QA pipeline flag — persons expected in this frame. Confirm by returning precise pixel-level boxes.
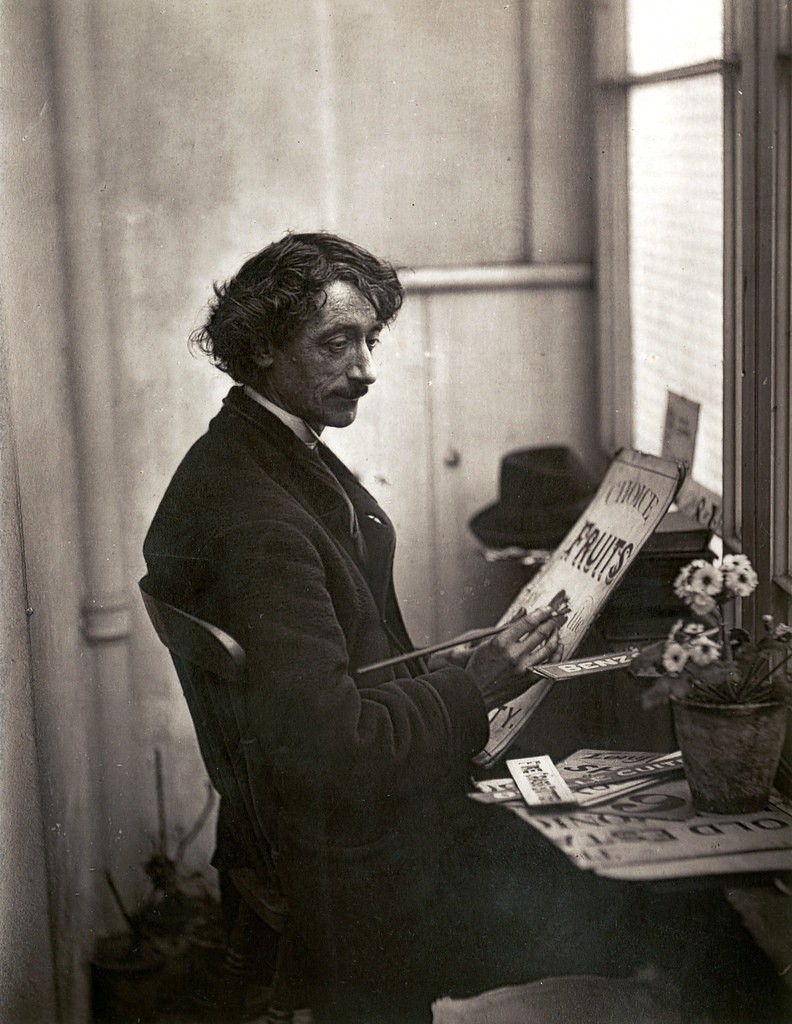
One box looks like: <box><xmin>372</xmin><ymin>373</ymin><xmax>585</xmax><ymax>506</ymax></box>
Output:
<box><xmin>141</xmin><ymin>229</ymin><xmax>792</xmax><ymax>1024</ymax></box>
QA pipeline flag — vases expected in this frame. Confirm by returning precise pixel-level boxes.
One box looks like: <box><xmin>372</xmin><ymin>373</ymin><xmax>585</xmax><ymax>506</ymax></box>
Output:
<box><xmin>668</xmin><ymin>688</ymin><xmax>788</xmax><ymax>815</ymax></box>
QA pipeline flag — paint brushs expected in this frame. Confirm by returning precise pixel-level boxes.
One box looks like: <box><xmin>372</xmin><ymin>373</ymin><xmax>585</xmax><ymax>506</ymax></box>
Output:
<box><xmin>355</xmin><ymin>608</ymin><xmax>573</xmax><ymax>674</ymax></box>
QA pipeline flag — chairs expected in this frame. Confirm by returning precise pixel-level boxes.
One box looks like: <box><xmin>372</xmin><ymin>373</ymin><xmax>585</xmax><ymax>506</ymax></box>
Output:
<box><xmin>138</xmin><ymin>573</ymin><xmax>309</xmax><ymax>1023</ymax></box>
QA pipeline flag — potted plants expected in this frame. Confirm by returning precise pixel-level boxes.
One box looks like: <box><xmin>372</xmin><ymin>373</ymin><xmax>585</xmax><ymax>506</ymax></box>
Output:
<box><xmin>88</xmin><ymin>931</ymin><xmax>168</xmax><ymax>1024</ymax></box>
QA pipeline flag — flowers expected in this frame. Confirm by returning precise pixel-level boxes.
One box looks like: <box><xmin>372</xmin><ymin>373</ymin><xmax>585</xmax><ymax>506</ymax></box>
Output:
<box><xmin>626</xmin><ymin>551</ymin><xmax>792</xmax><ymax>712</ymax></box>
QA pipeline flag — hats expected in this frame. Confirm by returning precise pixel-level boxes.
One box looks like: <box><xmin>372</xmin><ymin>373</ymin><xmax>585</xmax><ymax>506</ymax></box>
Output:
<box><xmin>468</xmin><ymin>445</ymin><xmax>598</xmax><ymax>552</ymax></box>
